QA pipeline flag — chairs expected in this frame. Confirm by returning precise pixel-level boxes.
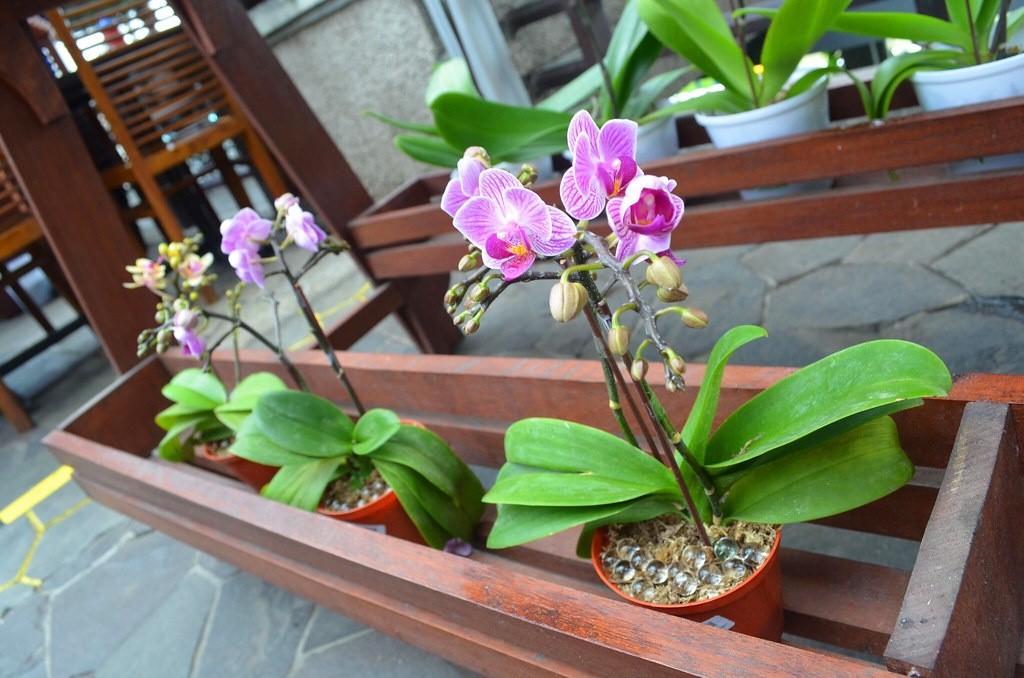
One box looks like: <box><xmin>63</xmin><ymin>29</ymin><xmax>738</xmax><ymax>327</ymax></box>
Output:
<box><xmin>0</xmin><ymin>0</ymin><xmax>289</xmax><ymax>445</ymax></box>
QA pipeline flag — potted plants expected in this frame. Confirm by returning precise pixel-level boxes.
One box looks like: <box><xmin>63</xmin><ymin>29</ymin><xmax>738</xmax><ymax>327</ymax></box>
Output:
<box><xmin>730</xmin><ymin>0</ymin><xmax>1023</xmax><ymax>176</ymax></box>
<box><xmin>636</xmin><ymin>0</ymin><xmax>852</xmax><ymax>198</ymax></box>
<box><xmin>434</xmin><ymin>0</ymin><xmax>703</xmax><ymax>217</ymax></box>
<box><xmin>363</xmin><ymin>52</ymin><xmax>611</xmax><ymax>193</ymax></box>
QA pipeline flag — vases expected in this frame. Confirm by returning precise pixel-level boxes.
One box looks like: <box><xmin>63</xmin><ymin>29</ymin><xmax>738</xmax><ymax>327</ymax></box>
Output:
<box><xmin>584</xmin><ymin>499</ymin><xmax>790</xmax><ymax>634</ymax></box>
<box><xmin>193</xmin><ymin>395</ymin><xmax>278</xmax><ymax>488</ymax></box>
<box><xmin>312</xmin><ymin>410</ymin><xmax>428</xmax><ymax>543</ymax></box>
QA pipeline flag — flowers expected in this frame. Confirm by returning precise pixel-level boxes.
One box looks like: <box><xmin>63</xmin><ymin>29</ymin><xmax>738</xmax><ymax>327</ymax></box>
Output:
<box><xmin>219</xmin><ymin>190</ymin><xmax>491</xmax><ymax>550</ymax></box>
<box><xmin>434</xmin><ymin>116</ymin><xmax>953</xmax><ymax>563</ymax></box>
<box><xmin>121</xmin><ymin>231</ymin><xmax>286</xmax><ymax>457</ymax></box>
